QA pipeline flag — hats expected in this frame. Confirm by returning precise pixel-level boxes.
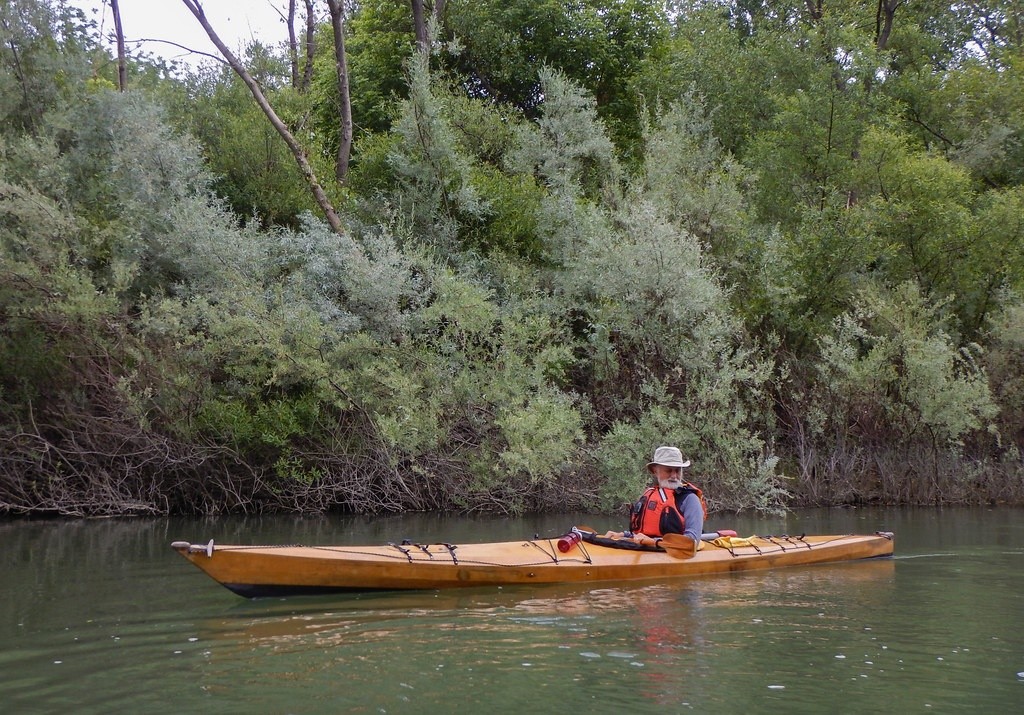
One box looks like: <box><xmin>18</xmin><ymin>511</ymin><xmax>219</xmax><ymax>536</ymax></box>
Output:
<box><xmin>646</xmin><ymin>446</ymin><xmax>691</xmax><ymax>474</ymax></box>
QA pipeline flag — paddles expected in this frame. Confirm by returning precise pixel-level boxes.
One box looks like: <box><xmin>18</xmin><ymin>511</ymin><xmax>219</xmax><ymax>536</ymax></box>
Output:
<box><xmin>573</xmin><ymin>524</ymin><xmax>697</xmax><ymax>562</ymax></box>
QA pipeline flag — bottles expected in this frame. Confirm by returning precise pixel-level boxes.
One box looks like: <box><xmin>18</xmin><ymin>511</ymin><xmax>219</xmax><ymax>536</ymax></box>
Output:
<box><xmin>557</xmin><ymin>526</ymin><xmax>583</xmax><ymax>553</ymax></box>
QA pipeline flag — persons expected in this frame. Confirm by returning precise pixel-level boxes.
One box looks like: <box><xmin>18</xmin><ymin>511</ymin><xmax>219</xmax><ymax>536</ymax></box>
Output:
<box><xmin>605</xmin><ymin>446</ymin><xmax>704</xmax><ymax>549</ymax></box>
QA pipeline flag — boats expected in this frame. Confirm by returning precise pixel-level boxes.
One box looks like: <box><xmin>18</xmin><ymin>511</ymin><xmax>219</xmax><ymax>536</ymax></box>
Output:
<box><xmin>171</xmin><ymin>532</ymin><xmax>896</xmax><ymax>599</ymax></box>
